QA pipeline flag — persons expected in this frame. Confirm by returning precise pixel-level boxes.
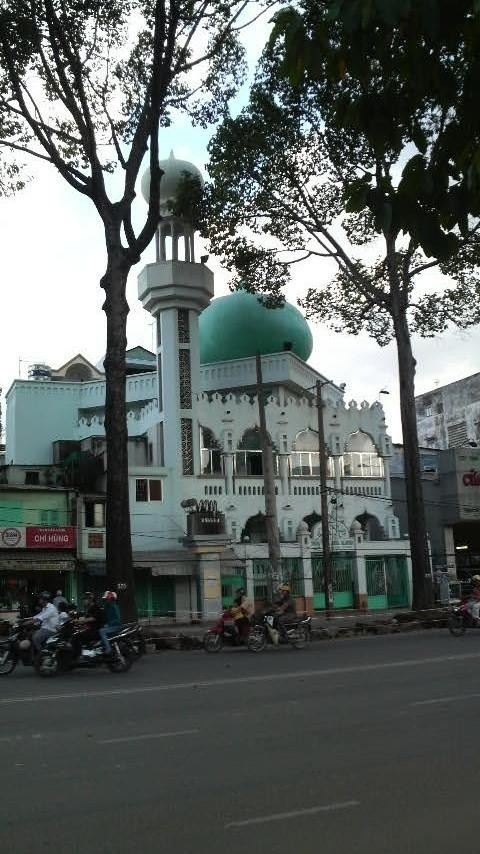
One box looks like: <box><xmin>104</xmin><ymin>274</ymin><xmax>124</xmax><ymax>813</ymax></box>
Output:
<box><xmin>230</xmin><ymin>589</ymin><xmax>254</xmax><ymax>638</ymax></box>
<box><xmin>272</xmin><ymin>582</ymin><xmax>297</xmax><ymax>642</ymax></box>
<box><xmin>468</xmin><ymin>575</ymin><xmax>480</xmax><ymax>622</ymax></box>
<box><xmin>24</xmin><ymin>590</ymin><xmax>122</xmax><ymax>655</ymax></box>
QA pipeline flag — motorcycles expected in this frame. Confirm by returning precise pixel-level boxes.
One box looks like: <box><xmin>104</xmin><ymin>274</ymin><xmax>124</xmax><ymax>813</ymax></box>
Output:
<box><xmin>202</xmin><ymin>605</ymin><xmax>311</xmax><ymax>653</ymax></box>
<box><xmin>0</xmin><ymin>612</ymin><xmax>144</xmax><ymax>676</ymax></box>
<box><xmin>448</xmin><ymin>597</ymin><xmax>480</xmax><ymax>635</ymax></box>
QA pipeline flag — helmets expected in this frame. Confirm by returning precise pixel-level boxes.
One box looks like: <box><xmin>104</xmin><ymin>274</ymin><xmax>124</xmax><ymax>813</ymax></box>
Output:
<box><xmin>37</xmin><ymin>592</ymin><xmax>51</xmax><ymax>601</ymax></box>
<box><xmin>102</xmin><ymin>591</ymin><xmax>117</xmax><ymax>600</ymax></box>
<box><xmin>472</xmin><ymin>575</ymin><xmax>479</xmax><ymax>585</ymax></box>
<box><xmin>236</xmin><ymin>588</ymin><xmax>245</xmax><ymax>595</ymax></box>
<box><xmin>279</xmin><ymin>585</ymin><xmax>289</xmax><ymax>592</ymax></box>
